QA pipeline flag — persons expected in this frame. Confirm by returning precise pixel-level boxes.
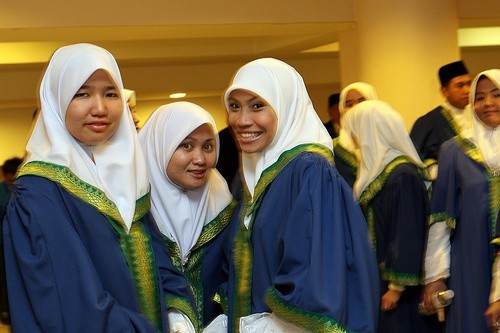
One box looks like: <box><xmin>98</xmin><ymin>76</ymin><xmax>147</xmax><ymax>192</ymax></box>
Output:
<box><xmin>202</xmin><ymin>58</ymin><xmax>381</xmax><ymax>333</ymax></box>
<box><xmin>137</xmin><ymin>101</ymin><xmax>237</xmax><ymax>333</ymax></box>
<box><xmin>123</xmin><ymin>88</ymin><xmax>140</xmax><ymax>130</ymax></box>
<box><xmin>216</xmin><ymin>127</ymin><xmax>239</xmax><ymax>192</ymax></box>
<box><xmin>324</xmin><ymin>93</ymin><xmax>340</xmax><ymax>139</ymax></box>
<box><xmin>332</xmin><ymin>82</ymin><xmax>437</xmax><ymax>333</ymax></box>
<box><xmin>2</xmin><ymin>43</ymin><xmax>169</xmax><ymax>333</ymax></box>
<box><xmin>409</xmin><ymin>61</ymin><xmax>472</xmax><ymax>162</ymax></box>
<box><xmin>0</xmin><ymin>157</ymin><xmax>24</xmax><ymax>322</ymax></box>
<box><xmin>422</xmin><ymin>69</ymin><xmax>500</xmax><ymax>333</ymax></box>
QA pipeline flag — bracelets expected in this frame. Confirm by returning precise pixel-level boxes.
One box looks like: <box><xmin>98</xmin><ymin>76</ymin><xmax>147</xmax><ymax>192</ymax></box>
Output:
<box><xmin>388</xmin><ymin>284</ymin><xmax>404</xmax><ymax>291</ymax></box>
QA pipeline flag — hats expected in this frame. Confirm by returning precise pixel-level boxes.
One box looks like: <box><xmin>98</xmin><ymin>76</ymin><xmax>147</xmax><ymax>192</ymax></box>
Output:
<box><xmin>328</xmin><ymin>94</ymin><xmax>339</xmax><ymax>106</ymax></box>
<box><xmin>439</xmin><ymin>60</ymin><xmax>467</xmax><ymax>84</ymax></box>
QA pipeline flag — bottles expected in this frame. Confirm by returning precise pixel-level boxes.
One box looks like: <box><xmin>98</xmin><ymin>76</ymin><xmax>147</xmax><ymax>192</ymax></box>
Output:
<box><xmin>416</xmin><ymin>289</ymin><xmax>455</xmax><ymax>314</ymax></box>
